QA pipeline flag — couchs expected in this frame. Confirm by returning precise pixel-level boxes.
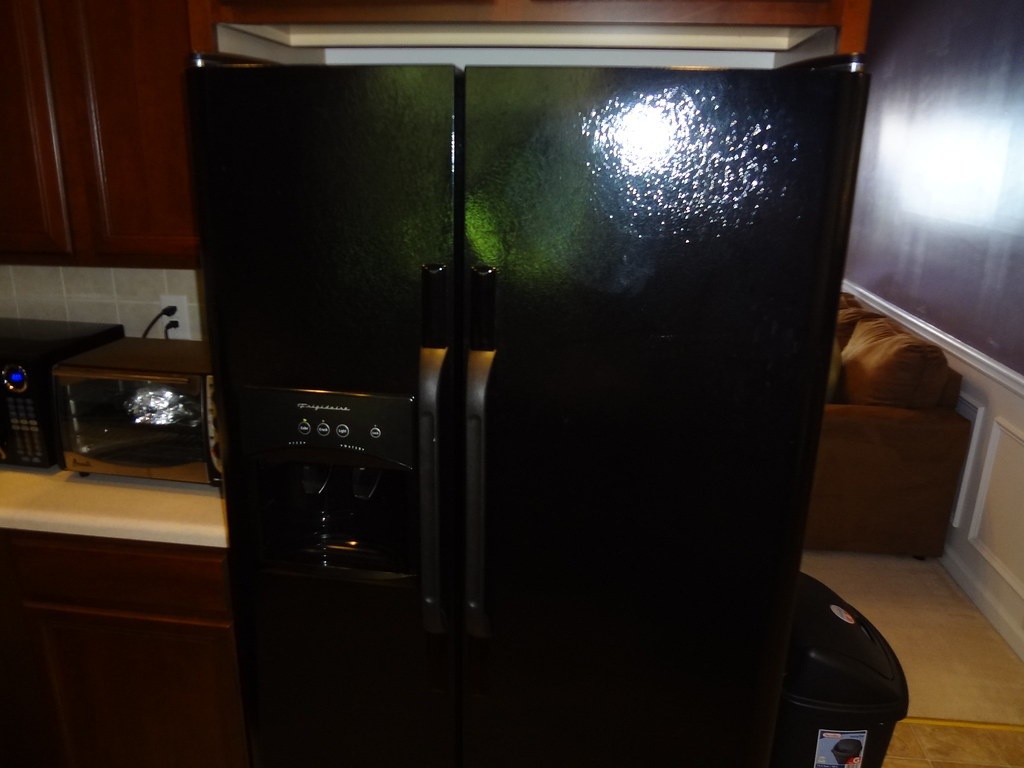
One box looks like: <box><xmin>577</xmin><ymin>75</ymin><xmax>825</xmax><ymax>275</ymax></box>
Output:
<box><xmin>805</xmin><ymin>293</ymin><xmax>977</xmax><ymax>557</ymax></box>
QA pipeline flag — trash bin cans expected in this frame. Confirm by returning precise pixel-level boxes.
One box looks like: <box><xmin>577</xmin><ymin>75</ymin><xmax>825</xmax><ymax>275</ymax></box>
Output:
<box><xmin>767</xmin><ymin>572</ymin><xmax>909</xmax><ymax>768</ymax></box>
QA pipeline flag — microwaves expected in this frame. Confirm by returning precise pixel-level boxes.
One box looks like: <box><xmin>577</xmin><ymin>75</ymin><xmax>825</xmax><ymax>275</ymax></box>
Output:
<box><xmin>0</xmin><ymin>317</ymin><xmax>125</xmax><ymax>471</ymax></box>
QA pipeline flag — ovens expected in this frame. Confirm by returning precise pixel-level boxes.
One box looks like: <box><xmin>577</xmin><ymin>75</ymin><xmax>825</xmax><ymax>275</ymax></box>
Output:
<box><xmin>51</xmin><ymin>336</ymin><xmax>223</xmax><ymax>486</ymax></box>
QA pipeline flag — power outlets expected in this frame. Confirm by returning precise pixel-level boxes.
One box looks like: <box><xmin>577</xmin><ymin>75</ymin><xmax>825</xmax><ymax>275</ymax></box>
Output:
<box><xmin>160</xmin><ymin>295</ymin><xmax>191</xmax><ymax>339</ymax></box>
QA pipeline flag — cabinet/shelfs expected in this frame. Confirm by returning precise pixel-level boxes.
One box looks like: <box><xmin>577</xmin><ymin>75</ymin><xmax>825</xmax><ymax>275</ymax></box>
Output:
<box><xmin>0</xmin><ymin>529</ymin><xmax>249</xmax><ymax>768</ymax></box>
<box><xmin>0</xmin><ymin>0</ymin><xmax>213</xmax><ymax>270</ymax></box>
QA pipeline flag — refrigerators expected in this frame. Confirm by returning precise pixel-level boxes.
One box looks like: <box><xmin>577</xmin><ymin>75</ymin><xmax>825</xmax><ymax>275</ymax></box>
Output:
<box><xmin>182</xmin><ymin>50</ymin><xmax>873</xmax><ymax>768</ymax></box>
